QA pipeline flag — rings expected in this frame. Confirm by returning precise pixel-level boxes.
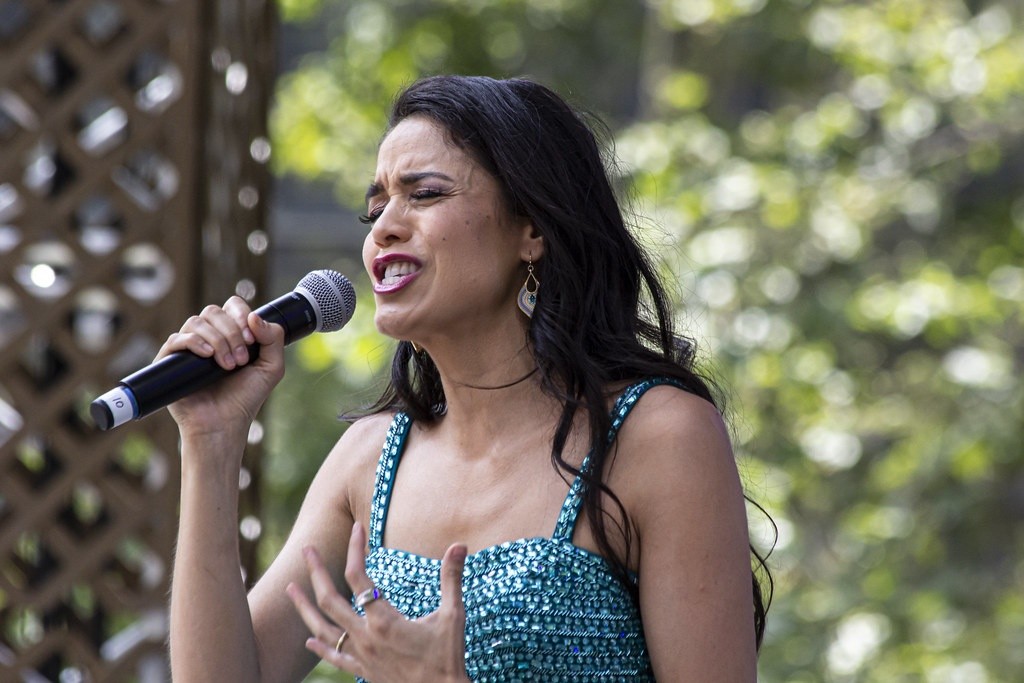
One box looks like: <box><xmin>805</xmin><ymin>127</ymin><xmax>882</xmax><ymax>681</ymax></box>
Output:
<box><xmin>356</xmin><ymin>587</ymin><xmax>382</xmax><ymax>607</ymax></box>
<box><xmin>335</xmin><ymin>631</ymin><xmax>347</xmax><ymax>653</ymax></box>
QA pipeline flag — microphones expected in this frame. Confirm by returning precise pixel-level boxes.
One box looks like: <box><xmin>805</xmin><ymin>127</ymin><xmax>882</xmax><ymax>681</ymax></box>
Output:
<box><xmin>91</xmin><ymin>268</ymin><xmax>357</xmax><ymax>431</ymax></box>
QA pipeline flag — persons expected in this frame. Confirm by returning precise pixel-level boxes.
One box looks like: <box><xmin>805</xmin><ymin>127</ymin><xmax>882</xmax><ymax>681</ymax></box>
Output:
<box><xmin>154</xmin><ymin>73</ymin><xmax>778</xmax><ymax>683</ymax></box>
